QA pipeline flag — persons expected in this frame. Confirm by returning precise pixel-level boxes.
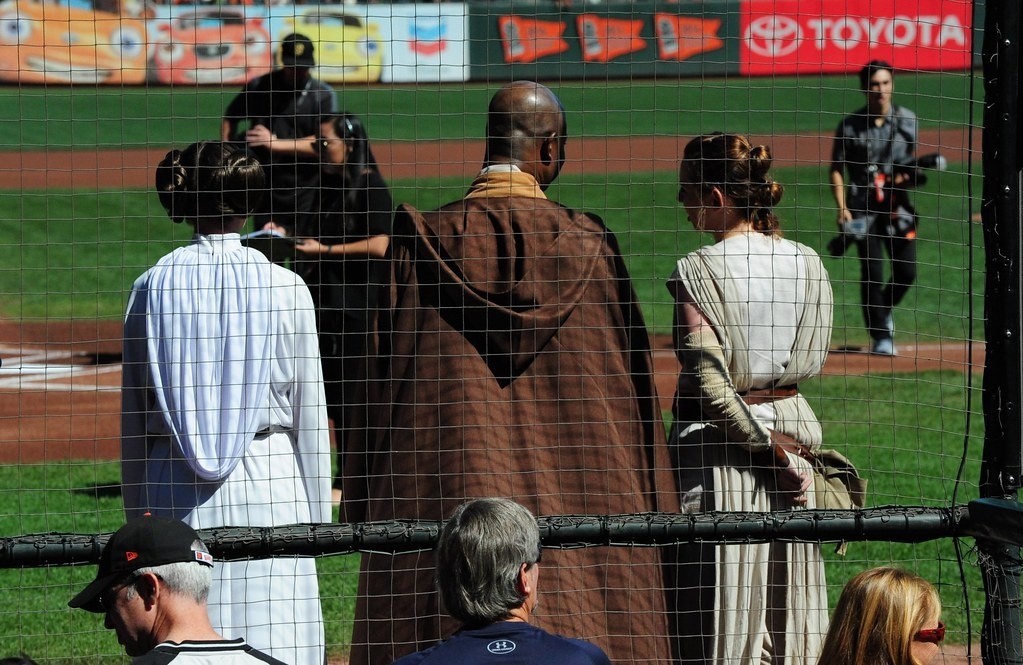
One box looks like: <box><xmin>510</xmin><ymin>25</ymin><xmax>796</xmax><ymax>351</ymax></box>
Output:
<box><xmin>68</xmin><ymin>512</ymin><xmax>285</xmax><ymax>665</ymax></box>
<box><xmin>339</xmin><ymin>81</ymin><xmax>680</xmax><ymax>665</ymax></box>
<box><xmin>819</xmin><ymin>567</ymin><xmax>942</xmax><ymax>665</ymax></box>
<box><xmin>220</xmin><ymin>33</ymin><xmax>335</xmax><ymax>238</ymax></box>
<box><xmin>666</xmin><ymin>130</ymin><xmax>834</xmax><ymax>665</ymax></box>
<box><xmin>265</xmin><ymin>113</ymin><xmax>393</xmax><ymax>524</ymax></box>
<box><xmin>120</xmin><ymin>141</ymin><xmax>331</xmax><ymax>665</ymax></box>
<box><xmin>830</xmin><ymin>59</ymin><xmax>920</xmax><ymax>357</ymax></box>
<box><xmin>393</xmin><ymin>496</ymin><xmax>613</xmax><ymax>664</ymax></box>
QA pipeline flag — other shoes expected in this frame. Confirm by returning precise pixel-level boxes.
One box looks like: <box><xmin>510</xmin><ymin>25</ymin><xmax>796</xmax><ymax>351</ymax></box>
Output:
<box><xmin>886</xmin><ymin>312</ymin><xmax>897</xmax><ymax>334</ymax></box>
<box><xmin>875</xmin><ymin>341</ymin><xmax>897</xmax><ymax>358</ymax></box>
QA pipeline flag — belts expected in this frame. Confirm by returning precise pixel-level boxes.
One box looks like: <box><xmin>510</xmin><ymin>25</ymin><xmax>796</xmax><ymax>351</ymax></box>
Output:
<box><xmin>741</xmin><ymin>383</ymin><xmax>798</xmax><ymax>404</ymax></box>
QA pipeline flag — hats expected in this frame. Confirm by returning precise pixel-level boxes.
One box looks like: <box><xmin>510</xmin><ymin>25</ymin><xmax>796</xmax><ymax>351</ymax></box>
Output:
<box><xmin>67</xmin><ymin>512</ymin><xmax>215</xmax><ymax>613</ymax></box>
<box><xmin>281</xmin><ymin>34</ymin><xmax>317</xmax><ymax>67</ymax></box>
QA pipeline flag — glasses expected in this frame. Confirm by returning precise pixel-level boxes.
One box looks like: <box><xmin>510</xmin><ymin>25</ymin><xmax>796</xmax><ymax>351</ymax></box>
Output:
<box><xmin>914</xmin><ymin>620</ymin><xmax>945</xmax><ymax>643</ymax></box>
<box><xmin>526</xmin><ymin>543</ymin><xmax>544</xmax><ymax>573</ymax></box>
<box><xmin>309</xmin><ymin>140</ymin><xmax>332</xmax><ymax>156</ymax></box>
<box><xmin>96</xmin><ymin>573</ymin><xmax>163</xmax><ymax>612</ymax></box>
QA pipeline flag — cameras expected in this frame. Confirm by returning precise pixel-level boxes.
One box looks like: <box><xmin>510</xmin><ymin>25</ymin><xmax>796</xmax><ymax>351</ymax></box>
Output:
<box><xmin>828</xmin><ymin>218</ymin><xmax>868</xmax><ymax>259</ymax></box>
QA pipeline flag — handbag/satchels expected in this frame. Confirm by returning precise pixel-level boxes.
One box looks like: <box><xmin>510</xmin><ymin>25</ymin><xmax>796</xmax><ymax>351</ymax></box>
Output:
<box><xmin>814</xmin><ymin>449</ymin><xmax>868</xmax><ymax>556</ymax></box>
